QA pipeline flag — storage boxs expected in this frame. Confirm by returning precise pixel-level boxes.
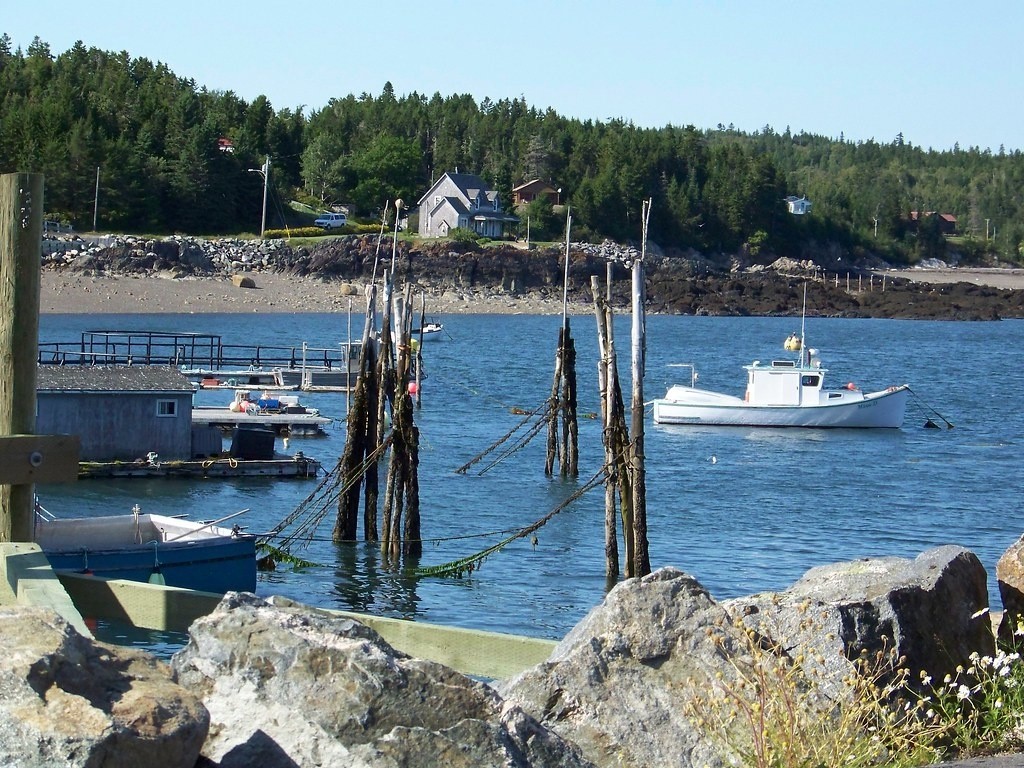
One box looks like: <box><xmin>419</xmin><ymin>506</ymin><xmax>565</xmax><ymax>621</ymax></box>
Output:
<box><xmin>258</xmin><ymin>396</ymin><xmax>307</xmax><ymax>415</ymax></box>
<box><xmin>202</xmin><ymin>379</ymin><xmax>221</xmax><ymax>385</ymax></box>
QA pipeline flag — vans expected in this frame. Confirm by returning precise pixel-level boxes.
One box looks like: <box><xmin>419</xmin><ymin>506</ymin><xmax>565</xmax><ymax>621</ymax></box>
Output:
<box><xmin>314</xmin><ymin>213</ymin><xmax>347</xmax><ymax>230</ymax></box>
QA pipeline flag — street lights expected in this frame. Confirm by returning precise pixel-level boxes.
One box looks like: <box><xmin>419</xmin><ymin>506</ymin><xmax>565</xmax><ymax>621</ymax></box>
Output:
<box><xmin>247</xmin><ymin>168</ymin><xmax>269</xmax><ymax>237</ymax></box>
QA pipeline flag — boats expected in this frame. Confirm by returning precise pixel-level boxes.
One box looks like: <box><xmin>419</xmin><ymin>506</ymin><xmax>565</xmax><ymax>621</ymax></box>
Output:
<box><xmin>36</xmin><ymin>503</ymin><xmax>258</xmax><ymax>595</ymax></box>
<box><xmin>652</xmin><ymin>281</ymin><xmax>911</xmax><ymax>430</ymax></box>
<box><xmin>376</xmin><ymin>316</ymin><xmax>445</xmax><ymax>343</ymax></box>
<box><xmin>182</xmin><ymin>335</ymin><xmax>430</xmax><ymax>436</ymax></box>
